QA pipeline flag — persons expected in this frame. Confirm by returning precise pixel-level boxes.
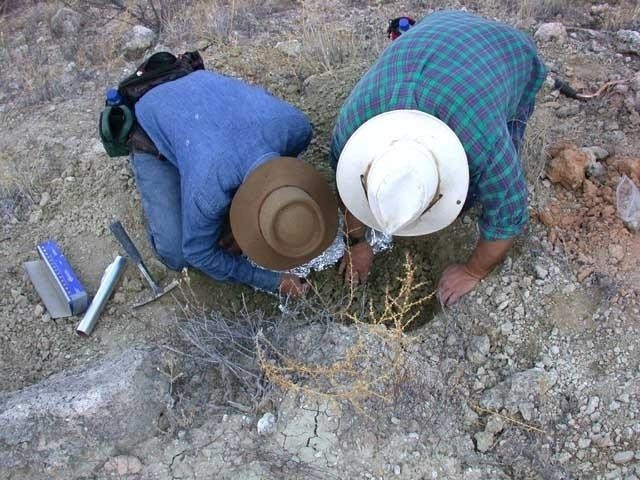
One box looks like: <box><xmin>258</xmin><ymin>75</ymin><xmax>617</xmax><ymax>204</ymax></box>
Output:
<box><xmin>329</xmin><ymin>9</ymin><xmax>545</xmax><ymax>307</ymax></box>
<box><xmin>126</xmin><ymin>53</ymin><xmax>315</xmax><ymax>301</ymax></box>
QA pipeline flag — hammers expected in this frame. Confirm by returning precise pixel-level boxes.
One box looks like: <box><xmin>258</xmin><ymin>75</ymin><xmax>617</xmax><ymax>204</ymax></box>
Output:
<box><xmin>109</xmin><ymin>221</ymin><xmax>179</xmax><ymax>309</ymax></box>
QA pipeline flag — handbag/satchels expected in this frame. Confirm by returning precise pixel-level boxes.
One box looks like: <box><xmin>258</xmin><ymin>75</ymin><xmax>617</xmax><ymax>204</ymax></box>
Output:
<box><xmin>98</xmin><ymin>49</ymin><xmax>205</xmax><ymax>162</ymax></box>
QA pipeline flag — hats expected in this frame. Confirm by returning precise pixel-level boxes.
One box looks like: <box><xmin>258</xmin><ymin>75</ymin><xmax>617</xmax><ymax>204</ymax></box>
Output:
<box><xmin>229</xmin><ymin>156</ymin><xmax>339</xmax><ymax>271</ymax></box>
<box><xmin>335</xmin><ymin>109</ymin><xmax>470</xmax><ymax>237</ymax></box>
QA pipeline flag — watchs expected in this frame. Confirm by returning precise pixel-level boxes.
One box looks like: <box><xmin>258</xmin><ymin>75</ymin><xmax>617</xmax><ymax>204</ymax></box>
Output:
<box><xmin>343</xmin><ymin>232</ymin><xmax>366</xmax><ymax>247</ymax></box>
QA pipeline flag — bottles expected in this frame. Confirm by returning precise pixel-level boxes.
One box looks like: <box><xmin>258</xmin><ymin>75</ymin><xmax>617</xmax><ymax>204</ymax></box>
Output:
<box><xmin>398</xmin><ymin>18</ymin><xmax>413</xmax><ymax>35</ymax></box>
<box><xmin>106</xmin><ymin>90</ymin><xmax>122</xmax><ymax>105</ymax></box>
